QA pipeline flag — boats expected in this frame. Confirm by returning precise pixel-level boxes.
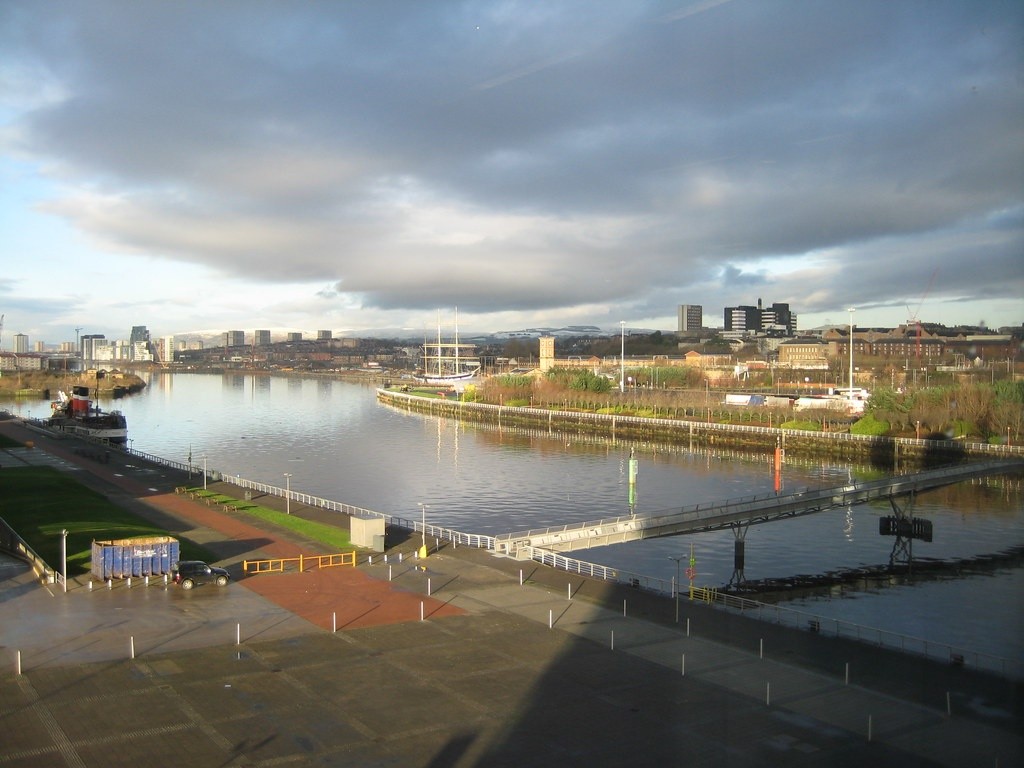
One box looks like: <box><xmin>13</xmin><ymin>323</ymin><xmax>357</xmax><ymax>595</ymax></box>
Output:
<box><xmin>42</xmin><ymin>371</ymin><xmax>128</xmax><ymax>444</ymax></box>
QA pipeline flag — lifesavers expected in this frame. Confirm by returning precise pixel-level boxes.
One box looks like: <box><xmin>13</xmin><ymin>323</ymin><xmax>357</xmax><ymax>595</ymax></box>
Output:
<box><xmin>685</xmin><ymin>568</ymin><xmax>695</xmax><ymax>579</ymax></box>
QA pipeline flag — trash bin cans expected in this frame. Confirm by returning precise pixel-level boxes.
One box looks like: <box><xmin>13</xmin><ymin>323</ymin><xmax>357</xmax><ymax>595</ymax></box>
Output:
<box><xmin>245</xmin><ymin>491</ymin><xmax>252</xmax><ymax>502</ymax></box>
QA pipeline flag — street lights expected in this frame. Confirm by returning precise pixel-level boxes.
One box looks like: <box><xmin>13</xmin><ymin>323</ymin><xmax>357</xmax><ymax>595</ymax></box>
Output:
<box><xmin>666</xmin><ymin>554</ymin><xmax>687</xmax><ymax>623</ymax></box>
<box><xmin>847</xmin><ymin>307</ymin><xmax>856</xmax><ymax>403</ymax></box>
<box><xmin>283</xmin><ymin>472</ymin><xmax>292</xmax><ymax>516</ymax></box>
<box><xmin>417</xmin><ymin>503</ymin><xmax>430</xmax><ymax>546</ymax></box>
<box><xmin>619</xmin><ymin>320</ymin><xmax>627</xmax><ymax>393</ymax></box>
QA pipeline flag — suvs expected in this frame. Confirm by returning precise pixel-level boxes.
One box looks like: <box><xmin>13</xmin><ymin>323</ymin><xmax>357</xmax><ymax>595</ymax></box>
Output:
<box><xmin>170</xmin><ymin>560</ymin><xmax>231</xmax><ymax>591</ymax></box>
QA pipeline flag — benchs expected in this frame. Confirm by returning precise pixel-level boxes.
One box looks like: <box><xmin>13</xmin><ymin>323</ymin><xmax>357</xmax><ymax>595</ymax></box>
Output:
<box><xmin>223</xmin><ymin>505</ymin><xmax>238</xmax><ymax>513</ymax></box>
<box><xmin>174</xmin><ymin>486</ymin><xmax>187</xmax><ymax>494</ymax></box>
<box><xmin>206</xmin><ymin>498</ymin><xmax>219</xmax><ymax>506</ymax></box>
<box><xmin>189</xmin><ymin>492</ymin><xmax>203</xmax><ymax>500</ymax></box>
